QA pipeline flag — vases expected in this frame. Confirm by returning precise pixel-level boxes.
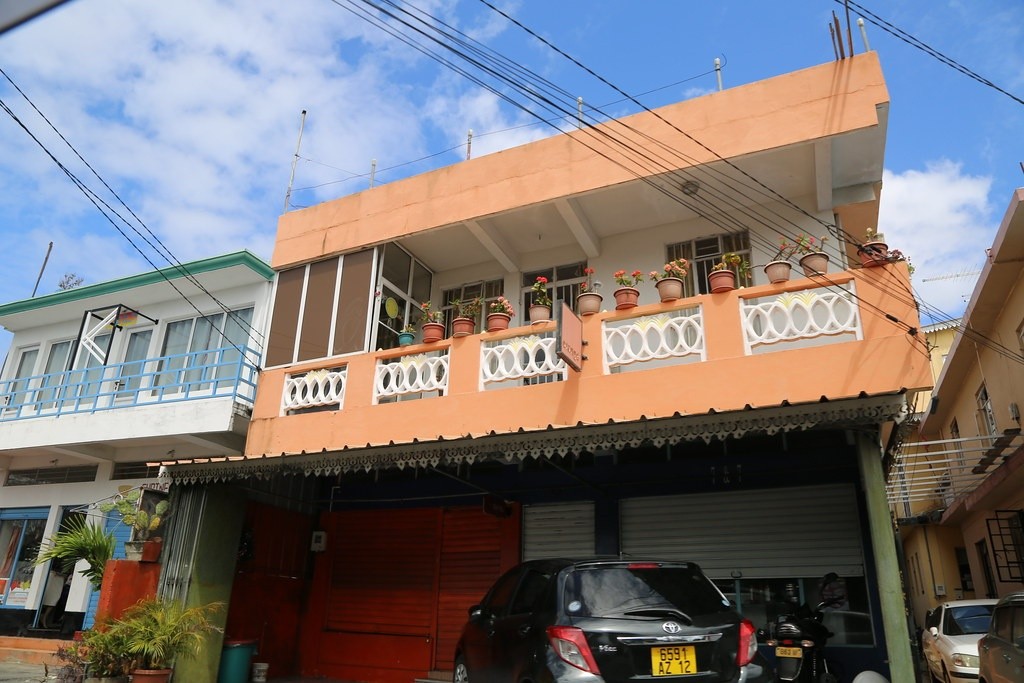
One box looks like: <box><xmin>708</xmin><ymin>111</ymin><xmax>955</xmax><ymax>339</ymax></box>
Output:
<box><xmin>613</xmin><ymin>287</ymin><xmax>640</xmax><ymax>310</ymax></box>
<box><xmin>487</xmin><ymin>313</ymin><xmax>511</xmax><ymax>332</ymax></box>
<box><xmin>764</xmin><ymin>261</ymin><xmax>792</xmax><ymax>284</ymax></box>
<box><xmin>577</xmin><ymin>293</ymin><xmax>604</xmax><ymax>316</ymax></box>
<box><xmin>857</xmin><ymin>242</ymin><xmax>888</xmax><ymax>269</ymax></box>
<box><xmin>799</xmin><ymin>252</ymin><xmax>830</xmax><ymax>278</ymax></box>
<box><xmin>708</xmin><ymin>270</ymin><xmax>735</xmax><ymax>294</ymax></box>
<box><xmin>529</xmin><ymin>305</ymin><xmax>551</xmax><ymax>326</ymax></box>
<box><xmin>452</xmin><ymin>316</ymin><xmax>476</xmax><ymax>339</ymax></box>
<box><xmin>422</xmin><ymin>324</ymin><xmax>445</xmax><ymax>344</ymax></box>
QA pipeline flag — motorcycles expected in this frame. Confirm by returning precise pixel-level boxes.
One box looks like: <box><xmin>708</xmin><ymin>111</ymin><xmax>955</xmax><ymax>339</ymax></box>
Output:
<box><xmin>766</xmin><ymin>606</ymin><xmax>832</xmax><ymax>681</ymax></box>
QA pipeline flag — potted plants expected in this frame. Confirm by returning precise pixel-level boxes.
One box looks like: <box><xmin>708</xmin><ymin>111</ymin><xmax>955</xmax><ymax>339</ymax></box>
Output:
<box><xmin>398</xmin><ymin>324</ymin><xmax>417</xmax><ymax>348</ymax></box>
<box><xmin>100</xmin><ymin>491</ymin><xmax>172</xmax><ymax>562</ymax></box>
<box><xmin>50</xmin><ymin>593</ymin><xmax>228</xmax><ymax>683</ymax></box>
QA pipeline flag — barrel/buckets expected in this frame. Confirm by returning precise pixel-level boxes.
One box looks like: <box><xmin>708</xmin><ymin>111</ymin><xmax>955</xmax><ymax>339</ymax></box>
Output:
<box><xmin>252</xmin><ymin>662</ymin><xmax>269</xmax><ymax>682</ymax></box>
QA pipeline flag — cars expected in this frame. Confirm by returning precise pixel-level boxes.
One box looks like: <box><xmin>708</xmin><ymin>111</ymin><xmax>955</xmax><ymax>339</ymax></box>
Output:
<box><xmin>921</xmin><ymin>598</ymin><xmax>1001</xmax><ymax>683</ymax></box>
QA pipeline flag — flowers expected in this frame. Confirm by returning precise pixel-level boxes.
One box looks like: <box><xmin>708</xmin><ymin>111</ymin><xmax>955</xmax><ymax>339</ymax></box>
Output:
<box><xmin>711</xmin><ymin>253</ymin><xmax>741</xmax><ymax>271</ymax></box>
<box><xmin>581</xmin><ymin>267</ymin><xmax>602</xmax><ymax>294</ymax></box>
<box><xmin>421</xmin><ymin>301</ymin><xmax>445</xmax><ymax>323</ymax></box>
<box><xmin>800</xmin><ymin>235</ymin><xmax>829</xmax><ymax>255</ymax></box>
<box><xmin>655</xmin><ymin>277</ymin><xmax>683</xmax><ymax>303</ymax></box>
<box><xmin>488</xmin><ymin>296</ymin><xmax>516</xmax><ymax>317</ymax></box>
<box><xmin>448</xmin><ymin>297</ymin><xmax>484</xmax><ymax>321</ymax></box>
<box><xmin>530</xmin><ymin>277</ymin><xmax>553</xmax><ymax>309</ymax></box>
<box><xmin>613</xmin><ymin>270</ymin><xmax>644</xmax><ymax>288</ymax></box>
<box><xmin>865</xmin><ymin>228</ymin><xmax>885</xmax><ymax>243</ymax></box>
<box><xmin>769</xmin><ymin>234</ymin><xmax>805</xmax><ymax>262</ymax></box>
<box><xmin>649</xmin><ymin>258</ymin><xmax>690</xmax><ymax>281</ymax></box>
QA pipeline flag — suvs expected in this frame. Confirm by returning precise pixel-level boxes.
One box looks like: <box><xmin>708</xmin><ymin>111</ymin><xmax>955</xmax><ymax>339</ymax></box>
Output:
<box><xmin>450</xmin><ymin>551</ymin><xmax>758</xmax><ymax>683</ymax></box>
<box><xmin>977</xmin><ymin>591</ymin><xmax>1024</xmax><ymax>683</ymax></box>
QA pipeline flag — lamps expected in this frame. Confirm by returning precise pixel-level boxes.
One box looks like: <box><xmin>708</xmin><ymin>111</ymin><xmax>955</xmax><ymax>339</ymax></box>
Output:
<box><xmin>682</xmin><ymin>181</ymin><xmax>700</xmax><ymax>196</ymax></box>
